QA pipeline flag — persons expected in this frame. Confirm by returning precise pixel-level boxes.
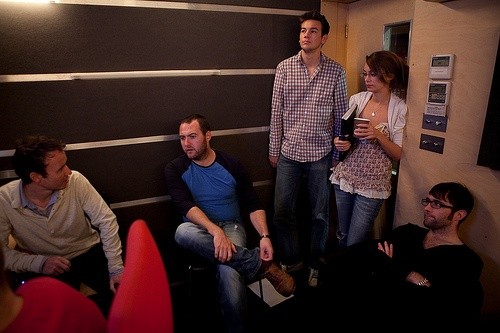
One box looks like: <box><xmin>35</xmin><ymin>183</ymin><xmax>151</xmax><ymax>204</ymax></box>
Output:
<box><xmin>334</xmin><ymin>182</ymin><xmax>482</xmax><ymax>333</ymax></box>
<box><xmin>162</xmin><ymin>108</ymin><xmax>294</xmax><ymax>332</ymax></box>
<box><xmin>0</xmin><ymin>128</ymin><xmax>126</xmax><ymax>311</ymax></box>
<box><xmin>326</xmin><ymin>50</ymin><xmax>409</xmax><ymax>284</ymax></box>
<box><xmin>269</xmin><ymin>11</ymin><xmax>350</xmax><ymax>287</ymax></box>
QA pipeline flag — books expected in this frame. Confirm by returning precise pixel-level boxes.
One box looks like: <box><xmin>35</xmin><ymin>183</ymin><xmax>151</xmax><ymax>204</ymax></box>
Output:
<box><xmin>338</xmin><ymin>104</ymin><xmax>360</xmax><ymax>161</ymax></box>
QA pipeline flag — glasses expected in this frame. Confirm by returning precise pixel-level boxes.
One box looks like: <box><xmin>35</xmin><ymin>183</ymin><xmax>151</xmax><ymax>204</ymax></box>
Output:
<box><xmin>360</xmin><ymin>71</ymin><xmax>387</xmax><ymax>77</ymax></box>
<box><xmin>421</xmin><ymin>198</ymin><xmax>453</xmax><ymax>209</ymax></box>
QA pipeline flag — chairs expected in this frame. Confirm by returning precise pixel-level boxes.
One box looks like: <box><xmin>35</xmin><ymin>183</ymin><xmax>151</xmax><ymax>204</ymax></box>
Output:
<box><xmin>182</xmin><ymin>250</ymin><xmax>263</xmax><ymax>300</ymax></box>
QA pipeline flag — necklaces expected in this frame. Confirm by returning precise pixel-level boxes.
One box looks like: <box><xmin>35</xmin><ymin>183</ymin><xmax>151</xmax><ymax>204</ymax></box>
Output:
<box><xmin>368</xmin><ymin>96</ymin><xmax>387</xmax><ymax>118</ymax></box>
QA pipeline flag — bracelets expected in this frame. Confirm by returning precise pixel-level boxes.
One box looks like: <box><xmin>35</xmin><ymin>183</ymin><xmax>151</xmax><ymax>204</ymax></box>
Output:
<box><xmin>418</xmin><ymin>278</ymin><xmax>429</xmax><ymax>286</ymax></box>
<box><xmin>259</xmin><ymin>234</ymin><xmax>269</xmax><ymax>239</ymax></box>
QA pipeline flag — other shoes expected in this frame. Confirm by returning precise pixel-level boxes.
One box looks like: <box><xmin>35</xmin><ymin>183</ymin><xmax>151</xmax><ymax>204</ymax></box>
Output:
<box><xmin>308</xmin><ymin>269</ymin><xmax>319</xmax><ymax>287</ymax></box>
<box><xmin>279</xmin><ymin>262</ymin><xmax>303</xmax><ymax>272</ymax></box>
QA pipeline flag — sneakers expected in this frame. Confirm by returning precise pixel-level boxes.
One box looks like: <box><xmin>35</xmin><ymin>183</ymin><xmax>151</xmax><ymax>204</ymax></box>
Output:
<box><xmin>263</xmin><ymin>263</ymin><xmax>296</xmax><ymax>297</ymax></box>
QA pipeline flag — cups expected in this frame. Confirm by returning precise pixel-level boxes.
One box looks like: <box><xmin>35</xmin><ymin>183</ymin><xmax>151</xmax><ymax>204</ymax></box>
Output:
<box><xmin>353</xmin><ymin>118</ymin><xmax>370</xmax><ymax>138</ymax></box>
